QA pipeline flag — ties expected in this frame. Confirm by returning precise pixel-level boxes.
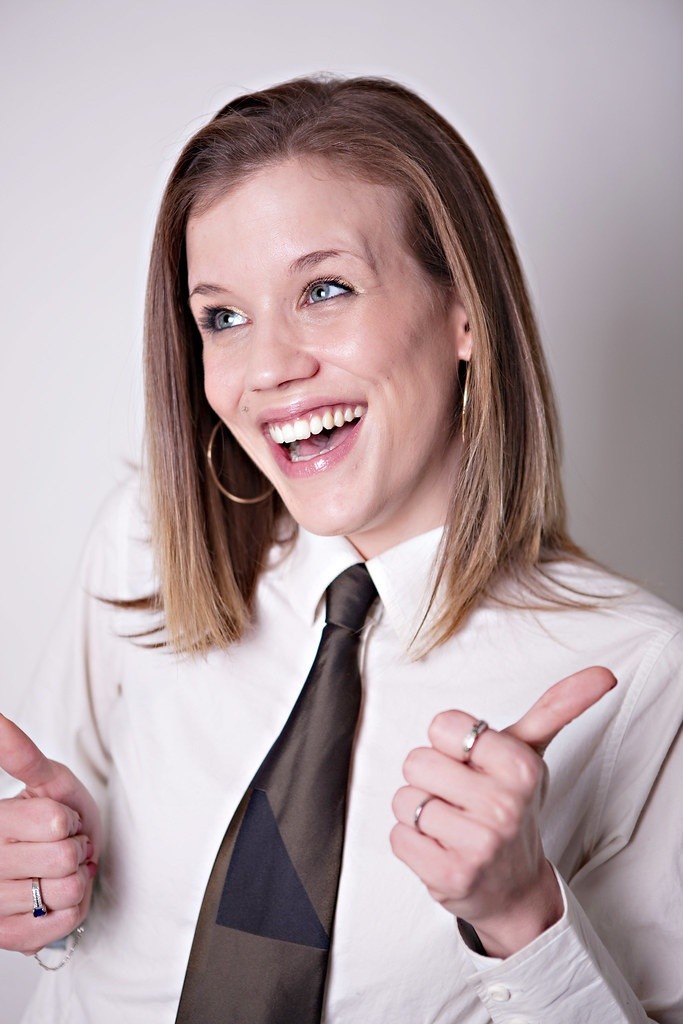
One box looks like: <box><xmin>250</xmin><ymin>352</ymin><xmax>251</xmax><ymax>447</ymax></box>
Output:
<box><xmin>174</xmin><ymin>562</ymin><xmax>380</xmax><ymax>1024</ymax></box>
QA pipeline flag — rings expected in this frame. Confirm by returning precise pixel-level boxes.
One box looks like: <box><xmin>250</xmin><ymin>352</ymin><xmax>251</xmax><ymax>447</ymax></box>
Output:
<box><xmin>461</xmin><ymin>718</ymin><xmax>488</xmax><ymax>763</ymax></box>
<box><xmin>414</xmin><ymin>795</ymin><xmax>436</xmax><ymax>835</ymax></box>
<box><xmin>31</xmin><ymin>877</ymin><xmax>47</xmax><ymax>919</ymax></box>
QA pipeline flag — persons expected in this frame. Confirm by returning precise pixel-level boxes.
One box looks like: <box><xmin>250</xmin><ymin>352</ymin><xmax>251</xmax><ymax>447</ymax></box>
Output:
<box><xmin>0</xmin><ymin>78</ymin><xmax>682</xmax><ymax>1024</ymax></box>
